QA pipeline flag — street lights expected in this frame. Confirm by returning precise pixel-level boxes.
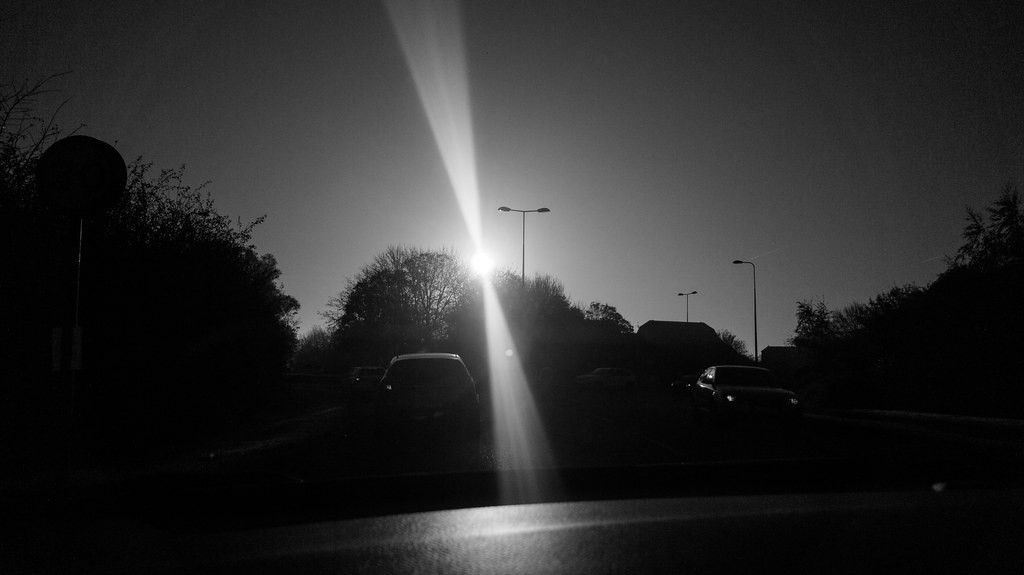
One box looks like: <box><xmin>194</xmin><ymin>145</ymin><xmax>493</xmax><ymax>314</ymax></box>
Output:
<box><xmin>732</xmin><ymin>259</ymin><xmax>758</xmax><ymax>366</ymax></box>
<box><xmin>498</xmin><ymin>205</ymin><xmax>551</xmax><ymax>285</ymax></box>
<box><xmin>676</xmin><ymin>290</ymin><xmax>698</xmax><ymax>323</ymax></box>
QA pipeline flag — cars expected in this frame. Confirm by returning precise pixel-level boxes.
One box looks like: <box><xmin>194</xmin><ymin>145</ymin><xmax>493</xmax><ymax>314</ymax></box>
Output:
<box><xmin>575</xmin><ymin>365</ymin><xmax>638</xmax><ymax>393</ymax></box>
<box><xmin>374</xmin><ymin>352</ymin><xmax>483</xmax><ymax>436</ymax></box>
<box><xmin>343</xmin><ymin>365</ymin><xmax>387</xmax><ymax>405</ymax></box>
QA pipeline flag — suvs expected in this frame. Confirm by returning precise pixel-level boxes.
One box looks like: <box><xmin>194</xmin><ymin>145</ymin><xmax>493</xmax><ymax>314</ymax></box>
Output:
<box><xmin>691</xmin><ymin>364</ymin><xmax>802</xmax><ymax>434</ymax></box>
